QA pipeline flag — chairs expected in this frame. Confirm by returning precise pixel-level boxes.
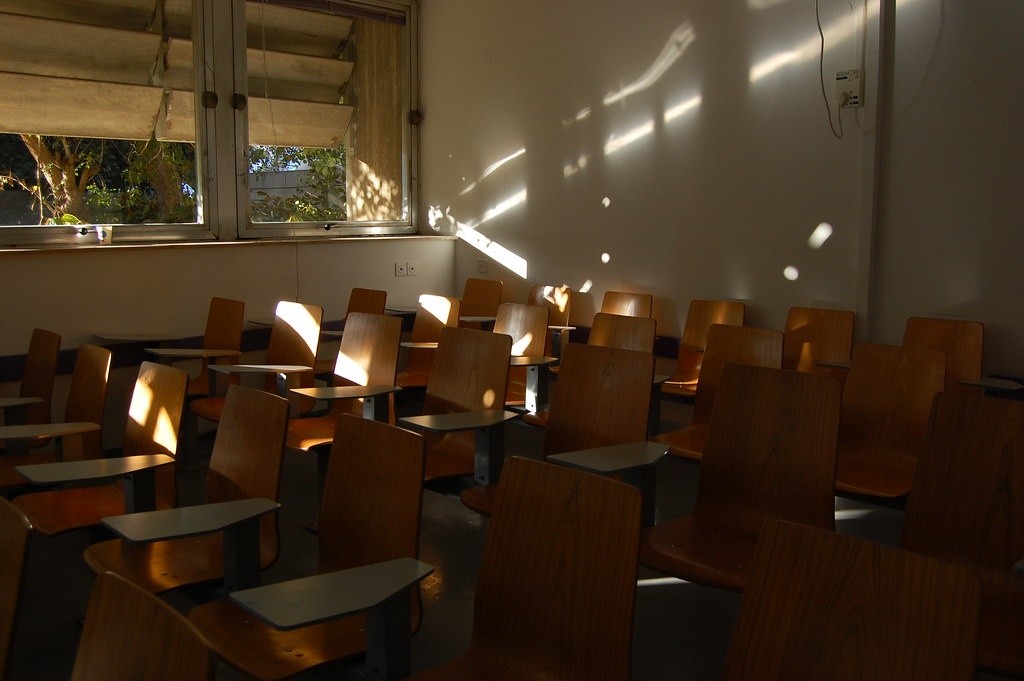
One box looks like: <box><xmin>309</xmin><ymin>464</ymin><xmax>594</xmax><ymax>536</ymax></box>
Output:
<box><xmin>1</xmin><ymin>343</ymin><xmax>114</xmax><ymax>491</ymax></box>
<box><xmin>1</xmin><ymin>329</ymin><xmax>62</xmax><ymax>458</ymax></box>
<box><xmin>12</xmin><ymin>362</ymin><xmax>189</xmax><ymax>542</ymax></box>
<box><xmin>73</xmin><ymin>279</ymin><xmax>1024</xmax><ymax>681</ymax></box>
<box><xmin>0</xmin><ymin>497</ymin><xmax>34</xmax><ymax>681</ymax></box>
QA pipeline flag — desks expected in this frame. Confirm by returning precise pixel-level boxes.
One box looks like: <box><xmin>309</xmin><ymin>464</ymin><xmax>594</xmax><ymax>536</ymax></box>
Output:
<box><xmin>1</xmin><ymin>308</ymin><xmax>681</xmax><ymax>630</ymax></box>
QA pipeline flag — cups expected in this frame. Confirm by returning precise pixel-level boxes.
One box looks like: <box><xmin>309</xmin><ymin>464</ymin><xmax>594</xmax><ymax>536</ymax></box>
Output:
<box><xmin>97</xmin><ymin>226</ymin><xmax>112</xmax><ymax>246</ymax></box>
